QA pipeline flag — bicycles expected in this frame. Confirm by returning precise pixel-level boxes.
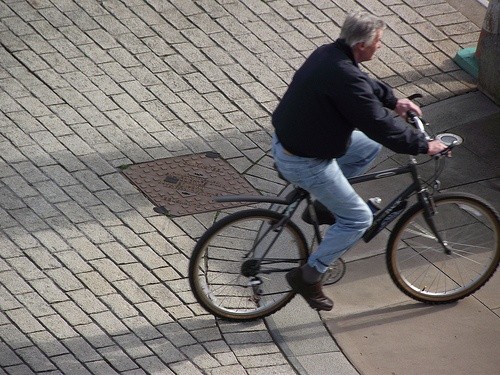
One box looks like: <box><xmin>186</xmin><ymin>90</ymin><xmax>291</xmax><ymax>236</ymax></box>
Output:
<box><xmin>187</xmin><ymin>93</ymin><xmax>500</xmax><ymax>323</ymax></box>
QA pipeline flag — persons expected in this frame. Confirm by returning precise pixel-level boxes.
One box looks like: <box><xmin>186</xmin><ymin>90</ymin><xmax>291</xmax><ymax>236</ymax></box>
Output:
<box><xmin>271</xmin><ymin>10</ymin><xmax>453</xmax><ymax>313</ymax></box>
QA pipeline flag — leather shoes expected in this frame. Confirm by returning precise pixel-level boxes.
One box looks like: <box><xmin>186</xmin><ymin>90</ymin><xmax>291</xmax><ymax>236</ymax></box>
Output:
<box><xmin>285</xmin><ymin>267</ymin><xmax>334</xmax><ymax>312</ymax></box>
<box><xmin>302</xmin><ymin>204</ymin><xmax>335</xmax><ymax>226</ymax></box>
